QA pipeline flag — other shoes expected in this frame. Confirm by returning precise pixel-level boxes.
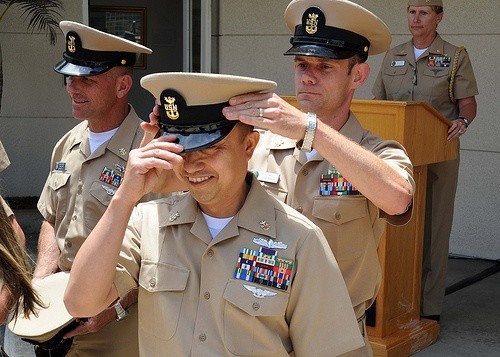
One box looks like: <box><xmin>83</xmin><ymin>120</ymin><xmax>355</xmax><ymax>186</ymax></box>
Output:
<box><xmin>423</xmin><ymin>315</ymin><xmax>440</xmax><ymax>321</ymax></box>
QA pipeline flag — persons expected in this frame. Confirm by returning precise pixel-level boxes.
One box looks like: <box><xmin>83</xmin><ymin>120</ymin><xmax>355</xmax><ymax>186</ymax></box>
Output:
<box><xmin>34</xmin><ymin>21</ymin><xmax>152</xmax><ymax>357</ymax></box>
<box><xmin>0</xmin><ymin>141</ymin><xmax>51</xmax><ymax>357</ymax></box>
<box><xmin>64</xmin><ymin>73</ymin><xmax>373</xmax><ymax>357</ymax></box>
<box><xmin>139</xmin><ymin>0</ymin><xmax>416</xmax><ymax>357</ymax></box>
<box><xmin>373</xmin><ymin>0</ymin><xmax>479</xmax><ymax>321</ymax></box>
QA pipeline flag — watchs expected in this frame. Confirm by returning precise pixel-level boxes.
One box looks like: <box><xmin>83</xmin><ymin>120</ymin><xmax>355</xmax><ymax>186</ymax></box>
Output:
<box><xmin>455</xmin><ymin>117</ymin><xmax>468</xmax><ymax>128</ymax></box>
<box><xmin>296</xmin><ymin>111</ymin><xmax>317</xmax><ymax>153</ymax></box>
<box><xmin>114</xmin><ymin>303</ymin><xmax>128</xmax><ymax>321</ymax></box>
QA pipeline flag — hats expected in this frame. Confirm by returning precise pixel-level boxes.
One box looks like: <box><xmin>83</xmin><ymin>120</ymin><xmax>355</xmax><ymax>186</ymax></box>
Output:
<box><xmin>284</xmin><ymin>0</ymin><xmax>392</xmax><ymax>60</ymax></box>
<box><xmin>141</xmin><ymin>71</ymin><xmax>278</xmax><ymax>154</ymax></box>
<box><xmin>7</xmin><ymin>271</ymin><xmax>83</xmax><ymax>357</ymax></box>
<box><xmin>54</xmin><ymin>19</ymin><xmax>152</xmax><ymax>77</ymax></box>
<box><xmin>407</xmin><ymin>0</ymin><xmax>443</xmax><ymax>7</ymax></box>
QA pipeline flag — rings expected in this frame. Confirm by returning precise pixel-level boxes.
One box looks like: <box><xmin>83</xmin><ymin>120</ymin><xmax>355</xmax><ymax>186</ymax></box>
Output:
<box><xmin>259</xmin><ymin>107</ymin><xmax>264</xmax><ymax>118</ymax></box>
